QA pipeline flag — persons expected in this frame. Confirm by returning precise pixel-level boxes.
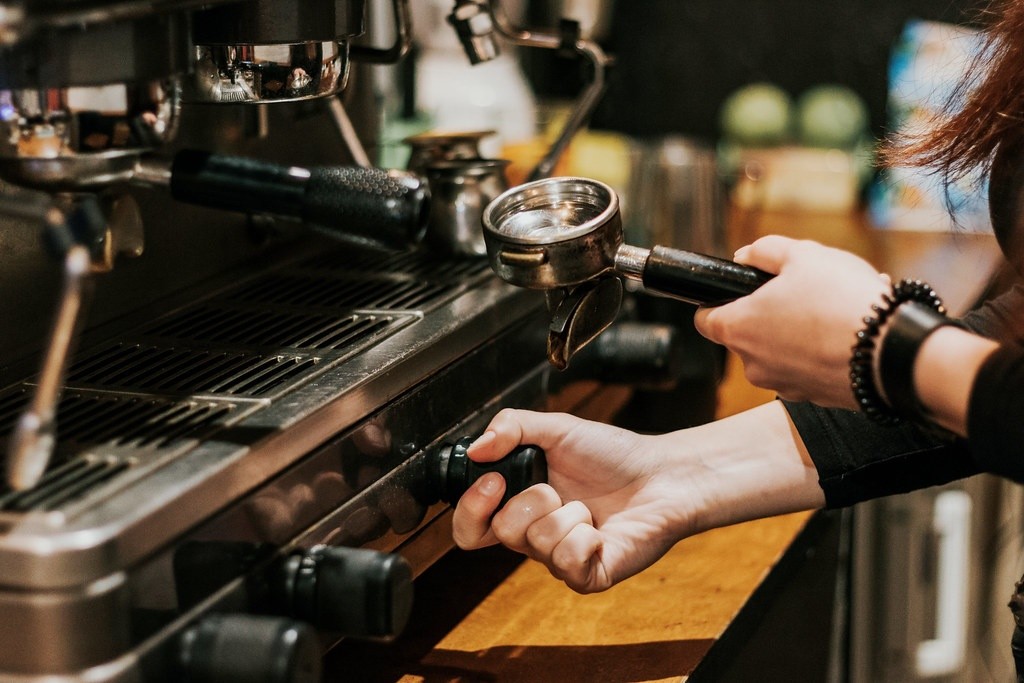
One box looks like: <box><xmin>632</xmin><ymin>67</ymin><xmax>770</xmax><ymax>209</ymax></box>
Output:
<box><xmin>451</xmin><ymin>0</ymin><xmax>1024</xmax><ymax>683</ymax></box>
<box><xmin>245</xmin><ymin>412</ymin><xmax>427</xmax><ymax>546</ymax></box>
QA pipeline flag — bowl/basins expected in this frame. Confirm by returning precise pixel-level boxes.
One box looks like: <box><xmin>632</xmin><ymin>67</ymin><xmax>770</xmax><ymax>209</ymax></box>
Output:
<box><xmin>501</xmin><ymin>130</ymin><xmax>630</xmax><ymax>211</ymax></box>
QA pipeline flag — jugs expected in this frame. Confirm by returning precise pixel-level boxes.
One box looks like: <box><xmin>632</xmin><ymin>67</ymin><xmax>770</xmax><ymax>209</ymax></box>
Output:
<box><xmin>630</xmin><ymin>135</ymin><xmax>743</xmax><ymax>259</ymax></box>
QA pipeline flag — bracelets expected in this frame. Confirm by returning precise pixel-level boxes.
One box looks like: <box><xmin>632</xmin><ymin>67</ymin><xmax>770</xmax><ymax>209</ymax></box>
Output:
<box><xmin>846</xmin><ymin>279</ymin><xmax>970</xmax><ymax>428</ymax></box>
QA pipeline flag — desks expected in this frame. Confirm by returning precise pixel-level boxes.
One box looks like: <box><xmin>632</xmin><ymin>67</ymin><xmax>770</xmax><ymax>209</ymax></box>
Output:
<box><xmin>391</xmin><ymin>202</ymin><xmax>1003</xmax><ymax>683</ymax></box>
